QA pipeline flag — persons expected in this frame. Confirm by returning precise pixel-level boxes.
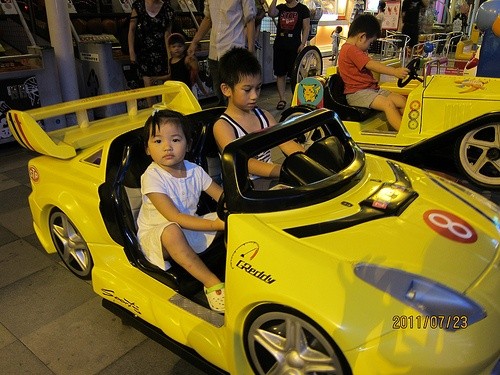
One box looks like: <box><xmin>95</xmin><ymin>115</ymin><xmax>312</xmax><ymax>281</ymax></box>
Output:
<box><xmin>268</xmin><ymin>0</ymin><xmax>311</xmax><ymax>109</ymax></box>
<box><xmin>128</xmin><ymin>0</ymin><xmax>178</xmax><ymax>89</ymax></box>
<box><xmin>136</xmin><ymin>109</ymin><xmax>226</xmax><ymax>315</ymax></box>
<box><xmin>212</xmin><ymin>47</ymin><xmax>305</xmax><ymax>190</ymax></box>
<box><xmin>187</xmin><ymin>0</ymin><xmax>257</xmax><ymax>105</ymax></box>
<box><xmin>402</xmin><ymin>0</ymin><xmax>434</xmax><ymax>49</ymax></box>
<box><xmin>329</xmin><ymin>26</ymin><xmax>342</xmax><ymax>61</ymax></box>
<box><xmin>166</xmin><ymin>32</ymin><xmax>198</xmax><ymax>90</ymax></box>
<box><xmin>337</xmin><ymin>15</ymin><xmax>410</xmax><ymax>131</ymax></box>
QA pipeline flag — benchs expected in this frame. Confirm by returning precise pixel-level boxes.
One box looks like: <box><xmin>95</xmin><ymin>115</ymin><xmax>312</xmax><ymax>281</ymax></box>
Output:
<box><xmin>325</xmin><ymin>73</ymin><xmax>382</xmax><ymax>122</ymax></box>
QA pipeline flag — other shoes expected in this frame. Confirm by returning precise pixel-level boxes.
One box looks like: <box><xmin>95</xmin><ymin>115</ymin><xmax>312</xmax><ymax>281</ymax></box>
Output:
<box><xmin>203</xmin><ymin>283</ymin><xmax>226</xmax><ymax>314</ymax></box>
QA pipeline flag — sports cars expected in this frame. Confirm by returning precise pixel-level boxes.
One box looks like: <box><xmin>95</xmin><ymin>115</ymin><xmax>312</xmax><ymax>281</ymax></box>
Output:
<box><xmin>7</xmin><ymin>81</ymin><xmax>500</xmax><ymax>375</ymax></box>
<box><xmin>289</xmin><ymin>45</ymin><xmax>500</xmax><ymax>194</ymax></box>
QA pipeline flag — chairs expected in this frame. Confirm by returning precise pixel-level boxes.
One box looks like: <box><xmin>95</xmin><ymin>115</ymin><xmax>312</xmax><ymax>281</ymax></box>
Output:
<box><xmin>114</xmin><ymin>111</ymin><xmax>248</xmax><ymax>295</ymax></box>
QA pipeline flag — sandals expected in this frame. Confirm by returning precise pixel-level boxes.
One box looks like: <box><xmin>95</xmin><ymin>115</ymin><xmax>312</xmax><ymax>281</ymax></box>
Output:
<box><xmin>277</xmin><ymin>100</ymin><xmax>286</xmax><ymax>110</ymax></box>
